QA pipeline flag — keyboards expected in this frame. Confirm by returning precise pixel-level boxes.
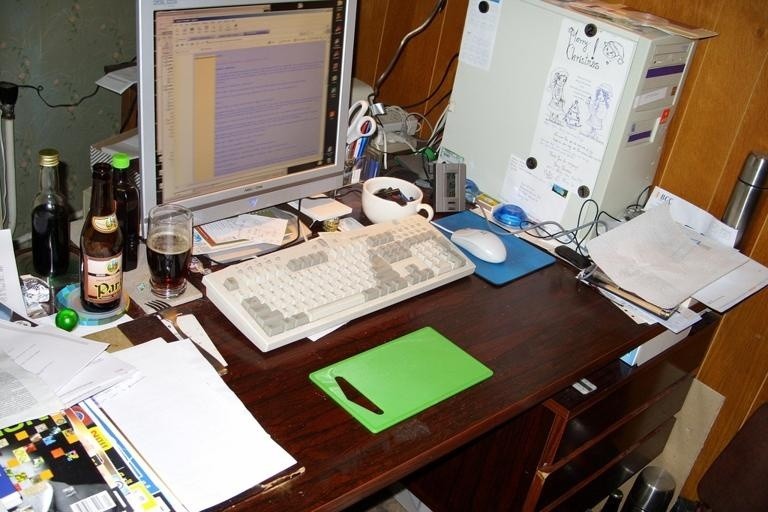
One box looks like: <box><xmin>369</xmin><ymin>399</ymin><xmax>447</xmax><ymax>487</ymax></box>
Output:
<box><xmin>201</xmin><ymin>213</ymin><xmax>476</xmax><ymax>352</ymax></box>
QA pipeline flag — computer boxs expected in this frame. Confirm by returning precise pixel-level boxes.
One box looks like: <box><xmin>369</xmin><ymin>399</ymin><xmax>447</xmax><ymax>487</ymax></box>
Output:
<box><xmin>437</xmin><ymin>0</ymin><xmax>698</xmax><ymax>256</ymax></box>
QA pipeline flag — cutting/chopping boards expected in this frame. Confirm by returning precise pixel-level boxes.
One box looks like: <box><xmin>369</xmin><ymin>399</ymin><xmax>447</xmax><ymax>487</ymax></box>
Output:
<box><xmin>309</xmin><ymin>326</ymin><xmax>493</xmax><ymax>436</ymax></box>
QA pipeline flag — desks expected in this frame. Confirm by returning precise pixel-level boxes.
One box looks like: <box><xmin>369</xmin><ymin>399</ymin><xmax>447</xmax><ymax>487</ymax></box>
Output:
<box><xmin>0</xmin><ymin>136</ymin><xmax>744</xmax><ymax>511</ymax></box>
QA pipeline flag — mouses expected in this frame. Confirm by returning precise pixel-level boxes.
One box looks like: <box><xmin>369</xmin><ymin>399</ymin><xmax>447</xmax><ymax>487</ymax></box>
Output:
<box><xmin>450</xmin><ymin>228</ymin><xmax>507</xmax><ymax>264</ymax></box>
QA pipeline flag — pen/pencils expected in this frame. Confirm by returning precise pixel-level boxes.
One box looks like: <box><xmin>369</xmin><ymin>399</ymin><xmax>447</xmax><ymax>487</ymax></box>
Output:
<box><xmin>354</xmin><ymin>137</ymin><xmax>362</xmax><ymax>158</ymax></box>
<box><xmin>350</xmin><ymin>121</ymin><xmax>368</xmax><ymax>158</ymax></box>
<box><xmin>357</xmin><ymin>122</ymin><xmax>372</xmax><ymax>158</ymax></box>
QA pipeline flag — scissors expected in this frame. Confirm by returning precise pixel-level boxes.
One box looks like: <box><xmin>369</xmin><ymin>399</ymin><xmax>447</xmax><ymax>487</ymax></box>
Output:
<box><xmin>347</xmin><ymin>101</ymin><xmax>376</xmax><ymax>145</ymax></box>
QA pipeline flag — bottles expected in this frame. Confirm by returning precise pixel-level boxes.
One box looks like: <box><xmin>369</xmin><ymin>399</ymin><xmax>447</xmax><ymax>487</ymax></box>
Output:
<box><xmin>619</xmin><ymin>466</ymin><xmax>674</xmax><ymax>512</ymax></box>
<box><xmin>599</xmin><ymin>488</ymin><xmax>624</xmax><ymax>512</ymax></box>
<box><xmin>31</xmin><ymin>149</ymin><xmax>70</xmax><ymax>278</ymax></box>
<box><xmin>79</xmin><ymin>163</ymin><xmax>121</xmax><ymax>314</ymax></box>
<box><xmin>112</xmin><ymin>153</ymin><xmax>140</xmax><ymax>273</ymax></box>
<box><xmin>721</xmin><ymin>152</ymin><xmax>767</xmax><ymax>243</ymax></box>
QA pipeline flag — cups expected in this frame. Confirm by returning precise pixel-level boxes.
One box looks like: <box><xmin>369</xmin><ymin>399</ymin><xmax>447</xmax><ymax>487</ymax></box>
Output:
<box><xmin>363</xmin><ymin>176</ymin><xmax>434</xmax><ymax>223</ymax></box>
<box><xmin>147</xmin><ymin>204</ymin><xmax>193</xmax><ymax>297</ymax></box>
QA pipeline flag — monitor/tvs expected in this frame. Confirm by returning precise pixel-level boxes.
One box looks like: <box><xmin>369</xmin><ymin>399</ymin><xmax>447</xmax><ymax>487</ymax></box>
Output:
<box><xmin>135</xmin><ymin>0</ymin><xmax>357</xmax><ymax>264</ymax></box>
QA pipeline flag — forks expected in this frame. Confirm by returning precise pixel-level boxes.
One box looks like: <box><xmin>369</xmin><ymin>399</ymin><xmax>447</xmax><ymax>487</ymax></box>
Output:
<box><xmin>144</xmin><ymin>299</ymin><xmax>229</xmax><ymax>377</ymax></box>
